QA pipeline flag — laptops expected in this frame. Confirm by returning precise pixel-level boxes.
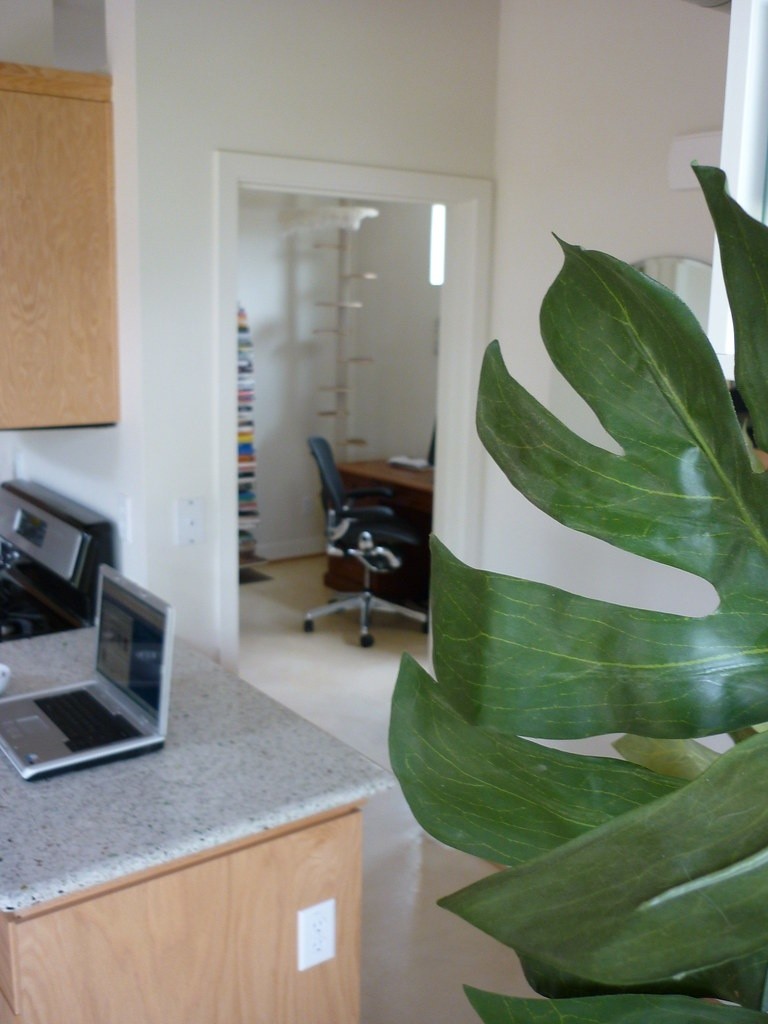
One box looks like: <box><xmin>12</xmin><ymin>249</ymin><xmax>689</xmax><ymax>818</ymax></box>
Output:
<box><xmin>0</xmin><ymin>564</ymin><xmax>174</xmax><ymax>781</ymax></box>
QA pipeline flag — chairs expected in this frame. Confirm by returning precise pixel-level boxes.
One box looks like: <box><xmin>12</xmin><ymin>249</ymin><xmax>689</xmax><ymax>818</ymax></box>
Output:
<box><xmin>302</xmin><ymin>436</ymin><xmax>429</xmax><ymax>646</ymax></box>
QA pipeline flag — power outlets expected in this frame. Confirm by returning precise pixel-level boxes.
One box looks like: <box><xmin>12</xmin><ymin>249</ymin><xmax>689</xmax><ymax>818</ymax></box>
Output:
<box><xmin>297</xmin><ymin>897</ymin><xmax>336</xmax><ymax>972</ymax></box>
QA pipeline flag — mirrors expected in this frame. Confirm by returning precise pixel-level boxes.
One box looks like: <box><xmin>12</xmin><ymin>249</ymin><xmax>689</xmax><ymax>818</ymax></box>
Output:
<box><xmin>629</xmin><ymin>255</ymin><xmax>713</xmax><ymax>335</ymax></box>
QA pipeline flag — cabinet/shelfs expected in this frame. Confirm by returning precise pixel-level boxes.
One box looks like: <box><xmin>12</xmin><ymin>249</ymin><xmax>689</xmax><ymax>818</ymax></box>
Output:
<box><xmin>237</xmin><ymin>303</ymin><xmax>273</xmax><ymax>584</ymax></box>
<box><xmin>0</xmin><ymin>796</ymin><xmax>365</xmax><ymax>1023</ymax></box>
<box><xmin>0</xmin><ymin>61</ymin><xmax>121</xmax><ymax>429</ymax></box>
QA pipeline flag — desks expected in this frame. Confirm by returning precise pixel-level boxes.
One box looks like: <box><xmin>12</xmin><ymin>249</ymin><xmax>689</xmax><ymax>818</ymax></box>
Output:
<box><xmin>323</xmin><ymin>457</ymin><xmax>435</xmax><ymax>607</ymax></box>
